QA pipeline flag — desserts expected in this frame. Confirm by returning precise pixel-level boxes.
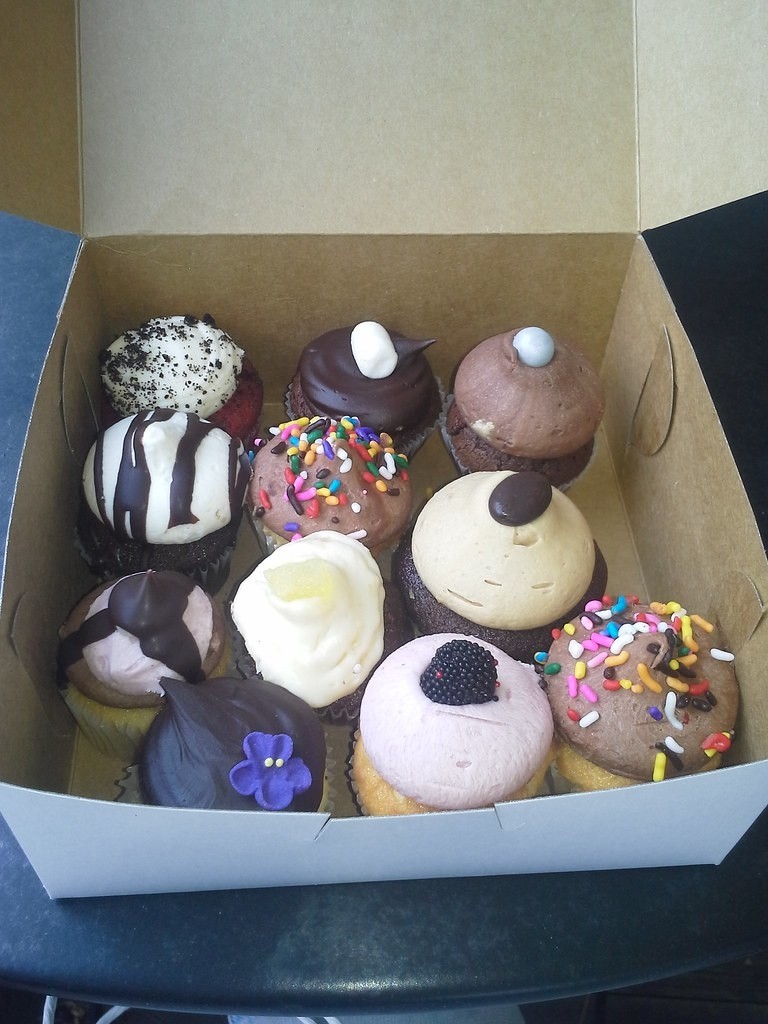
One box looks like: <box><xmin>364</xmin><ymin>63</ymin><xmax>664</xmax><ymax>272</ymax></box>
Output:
<box><xmin>51</xmin><ymin>310</ymin><xmax>739</xmax><ymax>817</ymax></box>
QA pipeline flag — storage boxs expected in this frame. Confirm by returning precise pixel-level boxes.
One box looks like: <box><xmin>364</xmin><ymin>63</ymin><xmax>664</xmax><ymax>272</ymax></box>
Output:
<box><xmin>0</xmin><ymin>0</ymin><xmax>768</xmax><ymax>899</ymax></box>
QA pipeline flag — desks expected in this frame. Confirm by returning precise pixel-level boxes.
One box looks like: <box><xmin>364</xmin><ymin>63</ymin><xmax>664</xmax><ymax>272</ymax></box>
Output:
<box><xmin>0</xmin><ymin>211</ymin><xmax>768</xmax><ymax>1024</ymax></box>
